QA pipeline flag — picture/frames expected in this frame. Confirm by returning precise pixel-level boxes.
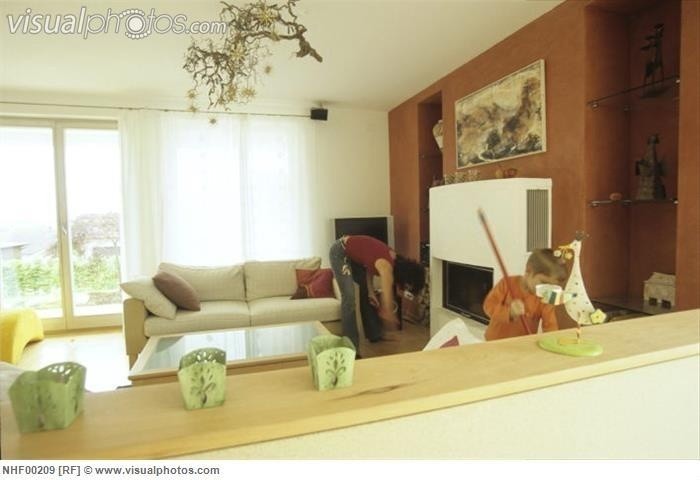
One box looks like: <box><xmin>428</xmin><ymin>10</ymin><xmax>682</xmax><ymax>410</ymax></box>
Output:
<box><xmin>453</xmin><ymin>57</ymin><xmax>547</xmax><ymax>170</ymax></box>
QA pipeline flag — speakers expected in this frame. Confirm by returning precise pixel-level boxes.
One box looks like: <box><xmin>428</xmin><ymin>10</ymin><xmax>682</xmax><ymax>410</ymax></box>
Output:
<box><xmin>311</xmin><ymin>108</ymin><xmax>329</xmax><ymax>120</ymax></box>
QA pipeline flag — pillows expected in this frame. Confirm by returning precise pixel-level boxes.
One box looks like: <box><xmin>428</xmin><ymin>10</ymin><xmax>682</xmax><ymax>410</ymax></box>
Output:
<box><xmin>291</xmin><ymin>269</ymin><xmax>332</xmax><ymax>299</ymax></box>
<box><xmin>152</xmin><ymin>273</ymin><xmax>199</xmax><ymax>311</ymax></box>
<box><xmin>121</xmin><ymin>277</ymin><xmax>176</xmax><ymax>321</ymax></box>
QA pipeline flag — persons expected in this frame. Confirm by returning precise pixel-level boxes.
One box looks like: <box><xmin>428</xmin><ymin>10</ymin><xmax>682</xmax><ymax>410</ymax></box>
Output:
<box><xmin>329</xmin><ymin>235</ymin><xmax>425</xmax><ymax>359</ymax></box>
<box><xmin>483</xmin><ymin>248</ymin><xmax>566</xmax><ymax>340</ymax></box>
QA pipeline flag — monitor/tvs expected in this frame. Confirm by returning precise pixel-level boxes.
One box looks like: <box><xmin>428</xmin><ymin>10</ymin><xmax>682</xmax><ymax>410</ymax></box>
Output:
<box><xmin>440</xmin><ymin>260</ymin><xmax>494</xmax><ymax>325</ymax></box>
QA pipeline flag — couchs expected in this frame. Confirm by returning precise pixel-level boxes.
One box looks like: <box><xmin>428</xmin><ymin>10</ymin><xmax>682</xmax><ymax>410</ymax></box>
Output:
<box><xmin>122</xmin><ymin>257</ymin><xmax>361</xmax><ymax>373</ymax></box>
